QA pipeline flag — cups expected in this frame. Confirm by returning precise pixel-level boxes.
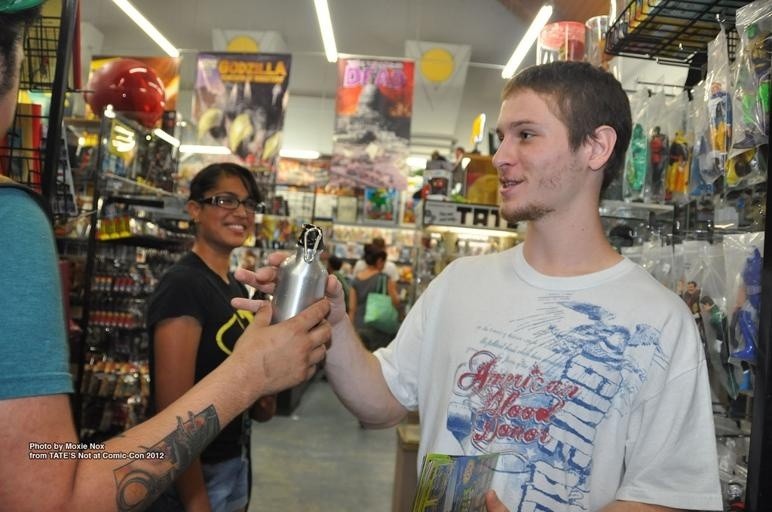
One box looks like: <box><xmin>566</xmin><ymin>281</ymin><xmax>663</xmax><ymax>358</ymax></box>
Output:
<box><xmin>269</xmin><ymin>224</ymin><xmax>329</xmax><ymax>324</ymax></box>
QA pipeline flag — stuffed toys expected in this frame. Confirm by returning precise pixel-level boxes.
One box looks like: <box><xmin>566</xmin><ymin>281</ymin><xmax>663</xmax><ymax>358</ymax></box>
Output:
<box><xmin>627</xmin><ymin>15</ymin><xmax>771</xmax><ymax>511</ymax></box>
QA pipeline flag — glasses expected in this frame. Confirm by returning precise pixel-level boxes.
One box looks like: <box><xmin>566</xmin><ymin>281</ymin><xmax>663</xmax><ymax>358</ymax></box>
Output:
<box><xmin>198</xmin><ymin>191</ymin><xmax>261</xmax><ymax>214</ymax></box>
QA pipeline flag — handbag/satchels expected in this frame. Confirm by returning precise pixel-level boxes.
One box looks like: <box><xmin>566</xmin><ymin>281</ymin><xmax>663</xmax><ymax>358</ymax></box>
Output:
<box><xmin>363</xmin><ymin>273</ymin><xmax>401</xmax><ymax>337</ymax></box>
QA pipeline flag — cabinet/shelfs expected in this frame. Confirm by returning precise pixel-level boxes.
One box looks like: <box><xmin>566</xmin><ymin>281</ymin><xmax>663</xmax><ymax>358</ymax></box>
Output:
<box><xmin>1</xmin><ymin>0</ymin><xmax>772</xmax><ymax>512</ymax></box>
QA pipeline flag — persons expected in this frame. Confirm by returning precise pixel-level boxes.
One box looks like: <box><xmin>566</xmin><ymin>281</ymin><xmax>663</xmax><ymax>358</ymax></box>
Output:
<box><xmin>138</xmin><ymin>160</ymin><xmax>267</xmax><ymax>511</ymax></box>
<box><xmin>627</xmin><ymin>15</ymin><xmax>771</xmax><ymax>511</ymax></box>
<box><xmin>230</xmin><ymin>59</ymin><xmax>725</xmax><ymax>512</ymax></box>
<box><xmin>0</xmin><ymin>0</ymin><xmax>333</xmax><ymax>512</ymax></box>
<box><xmin>326</xmin><ymin>232</ymin><xmax>441</xmax><ymax>351</ymax></box>
